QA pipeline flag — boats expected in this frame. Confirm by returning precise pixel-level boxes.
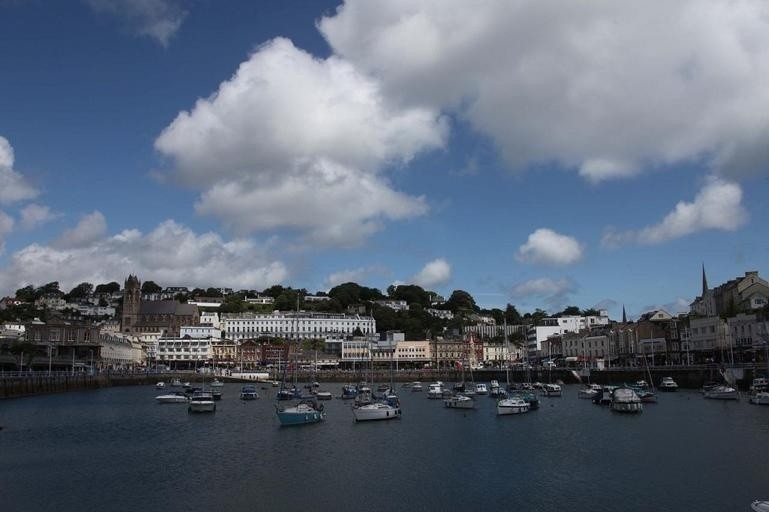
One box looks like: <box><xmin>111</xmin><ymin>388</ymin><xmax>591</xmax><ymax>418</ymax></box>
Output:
<box><xmin>273</xmin><ymin>293</ymin><xmax>332</xmax><ymax>424</ymax></box>
<box><xmin>156</xmin><ymin>377</ymin><xmax>260</xmax><ymax>416</ymax></box>
<box><xmin>660</xmin><ymin>377</ymin><xmax>679</xmax><ymax>391</ymax></box>
<box><xmin>580</xmin><ymin>380</ymin><xmax>657</xmax><ymax>412</ymax></box>
<box><xmin>412</xmin><ymin>381</ymin><xmax>423</xmax><ymax>392</ymax></box>
<box><xmin>698</xmin><ymin>384</ymin><xmax>739</xmax><ymax>399</ymax></box>
<box><xmin>427</xmin><ymin>383</ymin><xmax>452</xmax><ymax>399</ymax></box>
<box><xmin>749</xmin><ymin>378</ymin><xmax>769</xmax><ymax>393</ymax></box>
<box><xmin>403</xmin><ymin>383</ymin><xmax>411</xmax><ymax>388</ymax></box>
<box><xmin>750</xmin><ymin>391</ymin><xmax>769</xmax><ymax>404</ymax></box>
<box><xmin>444</xmin><ymin>395</ymin><xmax>473</xmax><ymax>409</ymax></box>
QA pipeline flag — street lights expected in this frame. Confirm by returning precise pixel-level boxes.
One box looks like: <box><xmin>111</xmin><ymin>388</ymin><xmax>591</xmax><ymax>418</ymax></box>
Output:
<box><xmin>410</xmin><ymin>359</ymin><xmax>412</xmax><ymax>371</ymax></box>
<box><xmin>89</xmin><ymin>349</ymin><xmax>93</xmax><ymax>372</ymax></box>
<box><xmin>48</xmin><ymin>347</ymin><xmax>55</xmax><ymax>374</ymax></box>
<box><xmin>426</xmin><ymin>339</ymin><xmax>435</xmax><ymax>368</ymax></box>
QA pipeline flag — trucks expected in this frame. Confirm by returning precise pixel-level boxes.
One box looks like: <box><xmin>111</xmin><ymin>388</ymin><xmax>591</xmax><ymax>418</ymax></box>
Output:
<box><xmin>424</xmin><ymin>362</ymin><xmax>435</xmax><ymax>368</ymax></box>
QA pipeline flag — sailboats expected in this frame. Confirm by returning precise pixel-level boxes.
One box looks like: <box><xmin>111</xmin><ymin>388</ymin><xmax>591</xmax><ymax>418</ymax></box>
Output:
<box><xmin>342</xmin><ymin>308</ymin><xmax>401</xmax><ymax>421</ymax></box>
<box><xmin>453</xmin><ymin>318</ymin><xmax>561</xmax><ymax>415</ymax></box>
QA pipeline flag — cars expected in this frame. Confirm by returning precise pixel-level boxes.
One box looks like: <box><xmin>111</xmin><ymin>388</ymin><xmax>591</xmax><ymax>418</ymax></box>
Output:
<box><xmin>157</xmin><ymin>364</ymin><xmax>170</xmax><ymax>372</ymax></box>
